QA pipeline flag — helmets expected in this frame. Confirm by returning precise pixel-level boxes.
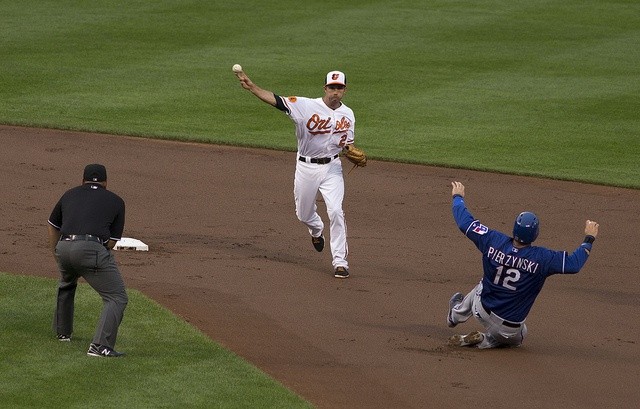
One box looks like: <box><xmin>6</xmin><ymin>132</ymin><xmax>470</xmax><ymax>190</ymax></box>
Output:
<box><xmin>513</xmin><ymin>212</ymin><xmax>539</xmax><ymax>244</ymax></box>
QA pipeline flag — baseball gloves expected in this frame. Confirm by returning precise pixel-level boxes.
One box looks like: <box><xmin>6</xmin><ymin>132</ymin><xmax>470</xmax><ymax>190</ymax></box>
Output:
<box><xmin>344</xmin><ymin>145</ymin><xmax>368</xmax><ymax>167</ymax></box>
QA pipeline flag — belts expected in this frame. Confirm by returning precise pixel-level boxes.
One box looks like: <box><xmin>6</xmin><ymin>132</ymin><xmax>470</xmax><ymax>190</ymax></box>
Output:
<box><xmin>58</xmin><ymin>234</ymin><xmax>105</xmax><ymax>244</ymax></box>
<box><xmin>299</xmin><ymin>153</ymin><xmax>339</xmax><ymax>164</ymax></box>
<box><xmin>480</xmin><ymin>295</ymin><xmax>520</xmax><ymax>328</ymax></box>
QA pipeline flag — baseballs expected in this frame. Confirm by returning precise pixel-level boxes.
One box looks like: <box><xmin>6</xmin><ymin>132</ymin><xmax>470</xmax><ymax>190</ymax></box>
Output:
<box><xmin>232</xmin><ymin>64</ymin><xmax>242</xmax><ymax>72</ymax></box>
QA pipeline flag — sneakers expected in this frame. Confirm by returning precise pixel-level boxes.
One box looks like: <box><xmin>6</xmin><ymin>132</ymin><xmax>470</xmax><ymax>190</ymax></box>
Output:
<box><xmin>447</xmin><ymin>292</ymin><xmax>464</xmax><ymax>328</ymax></box>
<box><xmin>311</xmin><ymin>233</ymin><xmax>326</xmax><ymax>252</ymax></box>
<box><xmin>334</xmin><ymin>266</ymin><xmax>350</xmax><ymax>278</ymax></box>
<box><xmin>448</xmin><ymin>331</ymin><xmax>484</xmax><ymax>347</ymax></box>
<box><xmin>87</xmin><ymin>342</ymin><xmax>125</xmax><ymax>358</ymax></box>
<box><xmin>55</xmin><ymin>332</ymin><xmax>71</xmax><ymax>342</ymax></box>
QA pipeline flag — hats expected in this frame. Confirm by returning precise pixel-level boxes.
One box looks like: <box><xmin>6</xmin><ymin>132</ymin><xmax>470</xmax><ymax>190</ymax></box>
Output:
<box><xmin>325</xmin><ymin>70</ymin><xmax>346</xmax><ymax>87</ymax></box>
<box><xmin>83</xmin><ymin>164</ymin><xmax>107</xmax><ymax>182</ymax></box>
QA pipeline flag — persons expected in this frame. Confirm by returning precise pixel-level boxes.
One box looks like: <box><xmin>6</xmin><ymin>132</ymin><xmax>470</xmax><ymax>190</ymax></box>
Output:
<box><xmin>47</xmin><ymin>164</ymin><xmax>128</xmax><ymax>359</ymax></box>
<box><xmin>234</xmin><ymin>69</ymin><xmax>367</xmax><ymax>279</ymax></box>
<box><xmin>447</xmin><ymin>180</ymin><xmax>600</xmax><ymax>350</ymax></box>
